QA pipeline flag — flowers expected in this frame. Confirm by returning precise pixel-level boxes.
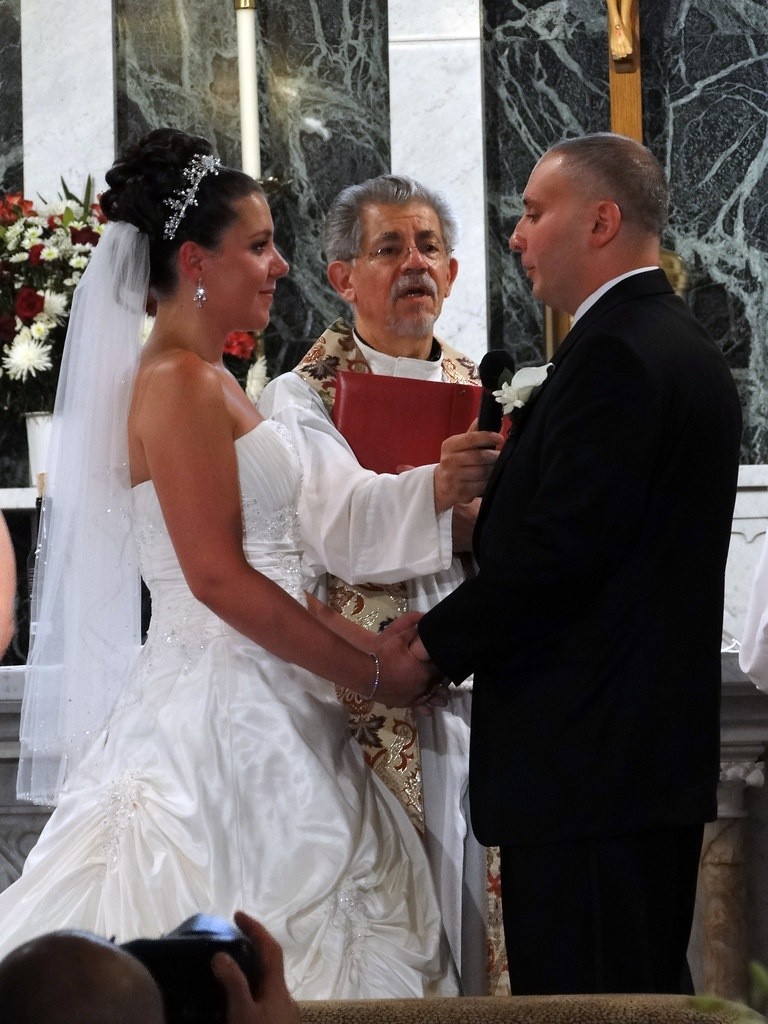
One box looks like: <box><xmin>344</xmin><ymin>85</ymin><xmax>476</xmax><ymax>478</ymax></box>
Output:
<box><xmin>0</xmin><ymin>178</ymin><xmax>259</xmax><ymax>415</ymax></box>
<box><xmin>491</xmin><ymin>360</ymin><xmax>554</xmax><ymax>414</ymax></box>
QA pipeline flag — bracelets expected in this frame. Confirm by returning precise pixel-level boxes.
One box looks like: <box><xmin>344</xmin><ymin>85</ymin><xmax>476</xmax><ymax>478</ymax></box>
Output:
<box><xmin>358</xmin><ymin>652</ymin><xmax>380</xmax><ymax>700</ymax></box>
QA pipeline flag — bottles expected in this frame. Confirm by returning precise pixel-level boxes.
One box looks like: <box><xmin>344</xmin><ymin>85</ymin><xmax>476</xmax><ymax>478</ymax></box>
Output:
<box><xmin>27</xmin><ymin>497</ymin><xmax>72</xmax><ymax>665</ymax></box>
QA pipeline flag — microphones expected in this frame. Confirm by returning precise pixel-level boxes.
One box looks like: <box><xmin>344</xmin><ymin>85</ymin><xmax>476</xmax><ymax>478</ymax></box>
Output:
<box><xmin>477</xmin><ymin>350</ymin><xmax>514</xmax><ymax>450</ymax></box>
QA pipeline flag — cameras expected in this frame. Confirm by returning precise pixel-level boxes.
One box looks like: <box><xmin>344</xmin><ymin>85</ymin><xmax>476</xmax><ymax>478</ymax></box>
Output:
<box><xmin>116</xmin><ymin>913</ymin><xmax>266</xmax><ymax>1024</ymax></box>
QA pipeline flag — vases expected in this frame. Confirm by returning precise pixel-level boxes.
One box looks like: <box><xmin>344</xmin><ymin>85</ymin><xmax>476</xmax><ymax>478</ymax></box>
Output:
<box><xmin>25</xmin><ymin>412</ymin><xmax>54</xmax><ymax>483</ymax></box>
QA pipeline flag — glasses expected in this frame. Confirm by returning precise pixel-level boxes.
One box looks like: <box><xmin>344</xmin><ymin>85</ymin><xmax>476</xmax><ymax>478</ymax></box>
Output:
<box><xmin>348</xmin><ymin>240</ymin><xmax>454</xmax><ymax>266</ymax></box>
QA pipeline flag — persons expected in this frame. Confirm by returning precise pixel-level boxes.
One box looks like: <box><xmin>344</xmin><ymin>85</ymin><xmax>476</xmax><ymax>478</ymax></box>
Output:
<box><xmin>1</xmin><ymin>513</ymin><xmax>17</xmax><ymax>657</ymax></box>
<box><xmin>0</xmin><ymin>911</ymin><xmax>304</xmax><ymax>1024</ymax></box>
<box><xmin>15</xmin><ymin>128</ymin><xmax>744</xmax><ymax>994</ymax></box>
